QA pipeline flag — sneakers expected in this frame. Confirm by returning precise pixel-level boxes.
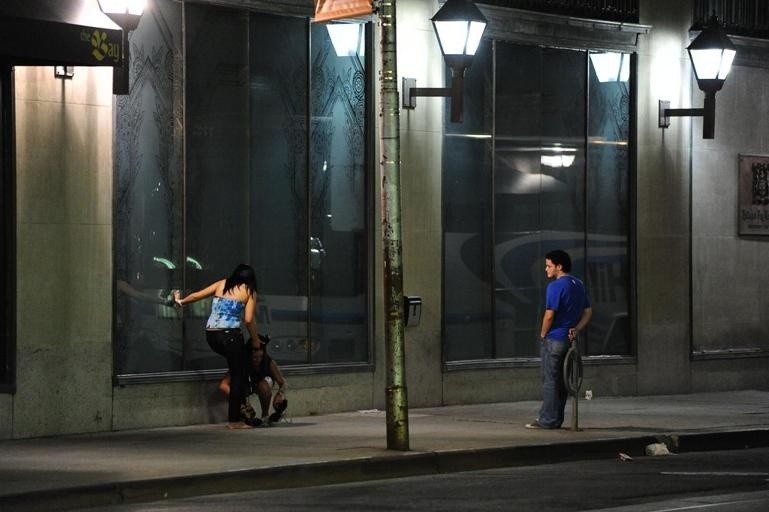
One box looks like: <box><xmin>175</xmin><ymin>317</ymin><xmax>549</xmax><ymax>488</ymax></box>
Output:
<box><xmin>525</xmin><ymin>420</ymin><xmax>560</xmax><ymax>429</ymax></box>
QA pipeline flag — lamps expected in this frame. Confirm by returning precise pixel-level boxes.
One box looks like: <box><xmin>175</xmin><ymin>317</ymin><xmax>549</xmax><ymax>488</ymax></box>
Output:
<box><xmin>98</xmin><ymin>1</ymin><xmax>147</xmax><ymax>95</ymax></box>
<box><xmin>591</xmin><ymin>51</ymin><xmax>631</xmax><ymax>82</ymax></box>
<box><xmin>403</xmin><ymin>1</ymin><xmax>486</xmax><ymax>122</ymax></box>
<box><xmin>325</xmin><ymin>24</ymin><xmax>366</xmax><ymax>59</ymax></box>
<box><xmin>658</xmin><ymin>9</ymin><xmax>737</xmax><ymax>137</ymax></box>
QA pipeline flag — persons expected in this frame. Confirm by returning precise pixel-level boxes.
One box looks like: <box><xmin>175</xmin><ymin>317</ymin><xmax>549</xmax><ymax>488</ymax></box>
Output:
<box><xmin>118</xmin><ymin>271</ymin><xmax>176</xmax><ymax>309</ymax></box>
<box><xmin>216</xmin><ymin>332</ymin><xmax>289</xmax><ymax>424</ymax></box>
<box><xmin>522</xmin><ymin>247</ymin><xmax>592</xmax><ymax>431</ymax></box>
<box><xmin>172</xmin><ymin>261</ymin><xmax>265</xmax><ymax>429</ymax></box>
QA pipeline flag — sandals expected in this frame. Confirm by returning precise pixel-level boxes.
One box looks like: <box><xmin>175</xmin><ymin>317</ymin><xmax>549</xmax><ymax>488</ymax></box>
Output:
<box><xmin>268</xmin><ymin>399</ymin><xmax>287</xmax><ymax>422</ymax></box>
<box><xmin>245</xmin><ymin>418</ymin><xmax>261</xmax><ymax>427</ymax></box>
